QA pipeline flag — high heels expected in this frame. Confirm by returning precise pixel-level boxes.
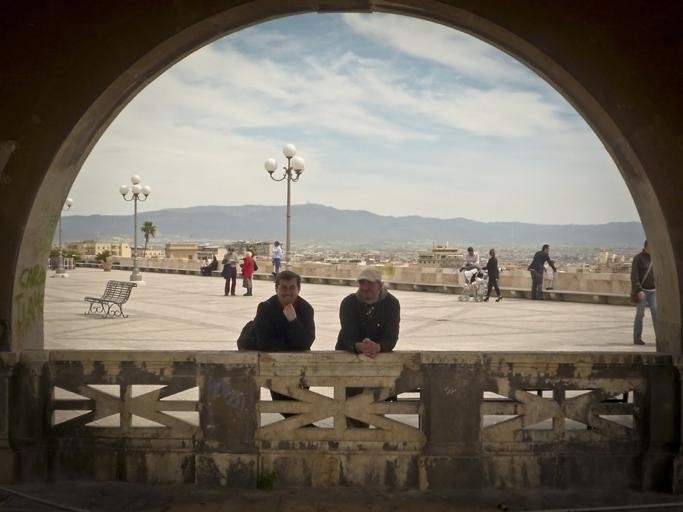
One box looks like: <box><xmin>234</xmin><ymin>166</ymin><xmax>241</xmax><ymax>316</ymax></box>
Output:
<box><xmin>495</xmin><ymin>296</ymin><xmax>502</xmax><ymax>302</ymax></box>
<box><xmin>483</xmin><ymin>296</ymin><xmax>489</xmax><ymax>301</ymax></box>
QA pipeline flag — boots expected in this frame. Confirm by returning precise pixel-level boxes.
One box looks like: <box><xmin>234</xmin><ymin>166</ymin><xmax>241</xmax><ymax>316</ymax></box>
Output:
<box><xmin>242</xmin><ymin>288</ymin><xmax>252</xmax><ymax>296</ymax></box>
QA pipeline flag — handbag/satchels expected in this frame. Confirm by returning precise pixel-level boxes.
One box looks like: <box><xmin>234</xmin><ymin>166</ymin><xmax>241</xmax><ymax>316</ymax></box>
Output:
<box><xmin>630</xmin><ymin>286</ymin><xmax>642</xmax><ymax>304</ymax></box>
<box><xmin>252</xmin><ymin>260</ymin><xmax>257</xmax><ymax>270</ymax></box>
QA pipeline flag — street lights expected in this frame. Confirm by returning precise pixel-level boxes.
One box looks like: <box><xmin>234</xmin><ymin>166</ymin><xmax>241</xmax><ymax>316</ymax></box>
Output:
<box><xmin>265</xmin><ymin>144</ymin><xmax>304</xmax><ymax>271</ymax></box>
<box><xmin>120</xmin><ymin>174</ymin><xmax>151</xmax><ymax>281</ymax></box>
<box><xmin>56</xmin><ymin>198</ymin><xmax>72</xmax><ymax>273</ymax></box>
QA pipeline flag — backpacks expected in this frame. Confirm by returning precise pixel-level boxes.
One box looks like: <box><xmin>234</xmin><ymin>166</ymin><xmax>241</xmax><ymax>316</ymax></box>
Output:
<box><xmin>236</xmin><ymin>320</ymin><xmax>256</xmax><ymax>351</ymax></box>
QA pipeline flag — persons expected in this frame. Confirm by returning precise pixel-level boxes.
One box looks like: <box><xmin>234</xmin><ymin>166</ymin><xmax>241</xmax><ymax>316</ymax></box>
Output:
<box><xmin>221</xmin><ymin>246</ymin><xmax>240</xmax><ymax>296</ymax></box>
<box><xmin>236</xmin><ymin>271</ymin><xmax>321</xmax><ymax>428</ymax></box>
<box><xmin>335</xmin><ymin>267</ymin><xmax>402</xmax><ymax>429</ymax></box>
<box><xmin>528</xmin><ymin>244</ymin><xmax>557</xmax><ymax>300</ymax></box>
<box><xmin>200</xmin><ymin>255</ymin><xmax>212</xmax><ymax>276</ymax></box>
<box><xmin>464</xmin><ymin>246</ymin><xmax>480</xmax><ymax>298</ymax></box>
<box><xmin>481</xmin><ymin>248</ymin><xmax>503</xmax><ymax>303</ymax></box>
<box><xmin>630</xmin><ymin>240</ymin><xmax>657</xmax><ymax>345</ymax></box>
<box><xmin>241</xmin><ymin>252</ymin><xmax>254</xmax><ymax>296</ymax></box>
<box><xmin>205</xmin><ymin>256</ymin><xmax>218</xmax><ymax>276</ymax></box>
<box><xmin>544</xmin><ymin>259</ymin><xmax>555</xmax><ymax>290</ymax></box>
<box><xmin>271</xmin><ymin>241</ymin><xmax>281</xmax><ymax>274</ymax></box>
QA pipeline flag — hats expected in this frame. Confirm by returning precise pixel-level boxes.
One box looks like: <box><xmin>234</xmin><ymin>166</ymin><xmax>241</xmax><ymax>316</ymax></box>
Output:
<box><xmin>355</xmin><ymin>268</ymin><xmax>383</xmax><ymax>284</ymax></box>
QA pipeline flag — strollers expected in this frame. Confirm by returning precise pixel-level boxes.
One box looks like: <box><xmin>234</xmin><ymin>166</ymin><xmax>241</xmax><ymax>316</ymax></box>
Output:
<box><xmin>458</xmin><ymin>263</ymin><xmax>489</xmax><ymax>302</ymax></box>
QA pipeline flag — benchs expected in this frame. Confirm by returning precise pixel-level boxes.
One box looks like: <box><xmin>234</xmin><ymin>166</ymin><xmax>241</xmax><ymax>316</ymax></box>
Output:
<box><xmin>83</xmin><ymin>280</ymin><xmax>137</xmax><ymax>319</ymax></box>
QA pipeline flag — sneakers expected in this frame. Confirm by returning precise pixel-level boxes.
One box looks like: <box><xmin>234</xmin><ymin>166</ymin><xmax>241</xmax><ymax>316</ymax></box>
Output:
<box><xmin>633</xmin><ymin>339</ymin><xmax>645</xmax><ymax>345</ymax></box>
